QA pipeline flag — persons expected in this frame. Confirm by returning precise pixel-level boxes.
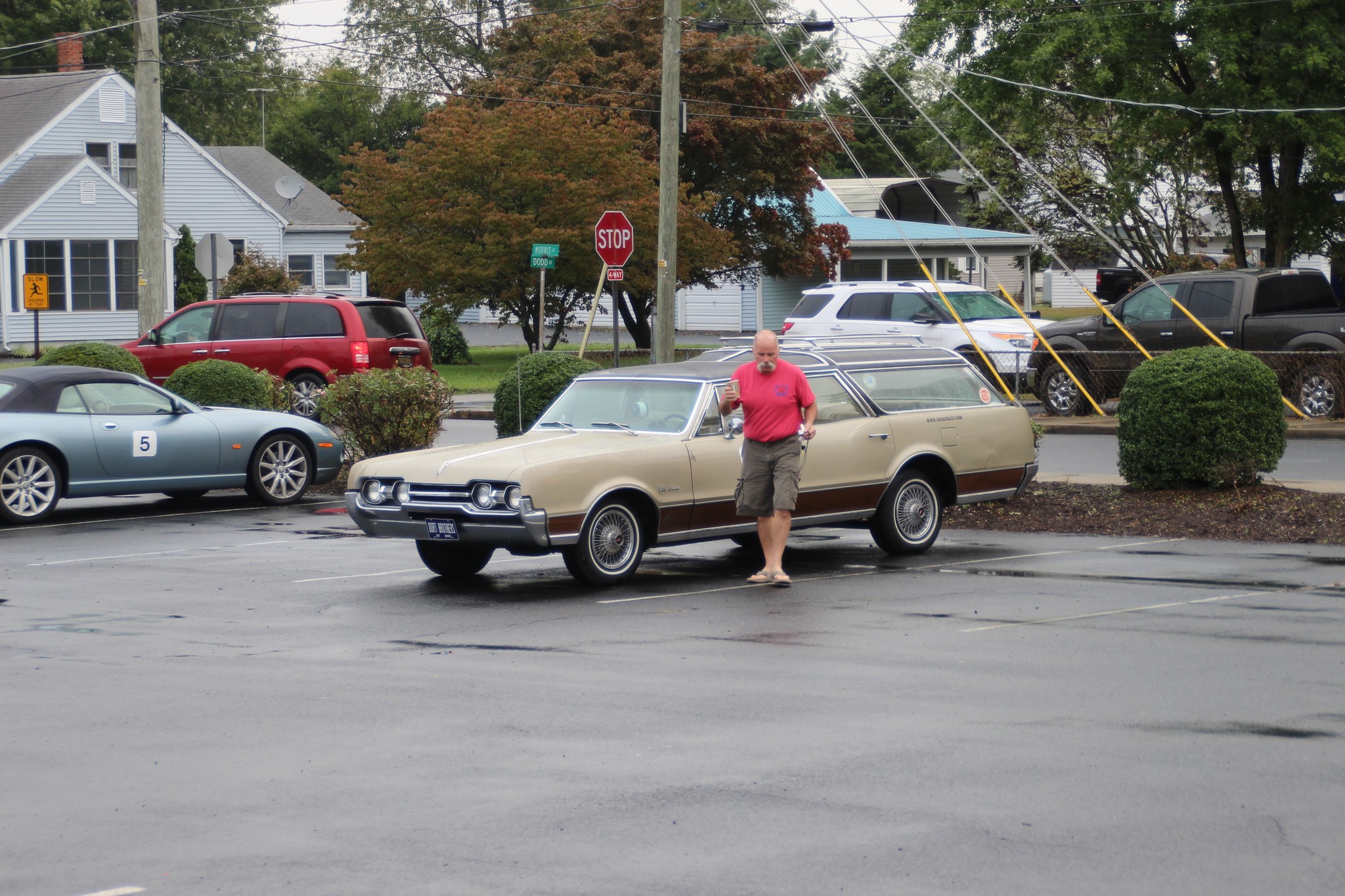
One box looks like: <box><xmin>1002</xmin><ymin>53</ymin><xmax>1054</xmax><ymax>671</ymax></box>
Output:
<box><xmin>718</xmin><ymin>331</ymin><xmax>818</xmax><ymax>580</ymax></box>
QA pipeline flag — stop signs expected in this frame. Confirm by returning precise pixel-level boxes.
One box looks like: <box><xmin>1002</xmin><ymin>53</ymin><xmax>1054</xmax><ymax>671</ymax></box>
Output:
<box><xmin>595</xmin><ymin>210</ymin><xmax>634</xmax><ymax>267</ymax></box>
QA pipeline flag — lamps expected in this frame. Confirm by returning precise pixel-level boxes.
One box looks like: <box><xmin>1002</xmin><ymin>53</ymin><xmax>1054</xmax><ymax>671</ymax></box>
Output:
<box><xmin>801</xmin><ymin>21</ymin><xmax>834</xmax><ymax>34</ymax></box>
<box><xmin>696</xmin><ymin>22</ymin><xmax>730</xmax><ymax>33</ymax></box>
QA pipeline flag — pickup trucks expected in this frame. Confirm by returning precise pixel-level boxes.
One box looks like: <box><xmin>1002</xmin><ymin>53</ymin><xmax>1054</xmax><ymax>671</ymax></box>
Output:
<box><xmin>1024</xmin><ymin>266</ymin><xmax>1345</xmax><ymax>422</ymax></box>
<box><xmin>1092</xmin><ymin>252</ymin><xmax>1258</xmax><ymax>307</ymax></box>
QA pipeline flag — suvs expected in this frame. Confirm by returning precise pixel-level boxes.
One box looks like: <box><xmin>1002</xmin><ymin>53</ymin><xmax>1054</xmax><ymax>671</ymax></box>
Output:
<box><xmin>344</xmin><ymin>334</ymin><xmax>1042</xmax><ymax>591</ymax></box>
<box><xmin>116</xmin><ymin>289</ymin><xmax>439</xmax><ymax>422</ymax></box>
<box><xmin>781</xmin><ymin>279</ymin><xmax>1062</xmax><ymax>394</ymax></box>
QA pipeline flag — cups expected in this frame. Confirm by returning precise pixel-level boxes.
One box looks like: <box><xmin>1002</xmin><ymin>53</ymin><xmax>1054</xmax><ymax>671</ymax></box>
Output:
<box><xmin>726</xmin><ymin>379</ymin><xmax>741</xmax><ymax>398</ymax></box>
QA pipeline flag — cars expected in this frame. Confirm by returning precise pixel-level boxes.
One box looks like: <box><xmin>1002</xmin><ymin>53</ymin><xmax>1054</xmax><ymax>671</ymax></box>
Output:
<box><xmin>0</xmin><ymin>364</ymin><xmax>346</xmax><ymax>526</ymax></box>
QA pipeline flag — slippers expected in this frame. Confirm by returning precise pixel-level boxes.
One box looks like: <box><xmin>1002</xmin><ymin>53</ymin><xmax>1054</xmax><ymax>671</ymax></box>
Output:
<box><xmin>747</xmin><ymin>571</ymin><xmax>769</xmax><ymax>583</ymax></box>
<box><xmin>769</xmin><ymin>571</ymin><xmax>791</xmax><ymax>584</ymax></box>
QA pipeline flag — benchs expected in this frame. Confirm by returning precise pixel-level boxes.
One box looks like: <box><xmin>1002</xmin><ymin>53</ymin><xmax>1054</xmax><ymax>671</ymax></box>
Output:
<box><xmin>825</xmin><ymin>412</ymin><xmax>860</xmax><ymax>420</ymax></box>
